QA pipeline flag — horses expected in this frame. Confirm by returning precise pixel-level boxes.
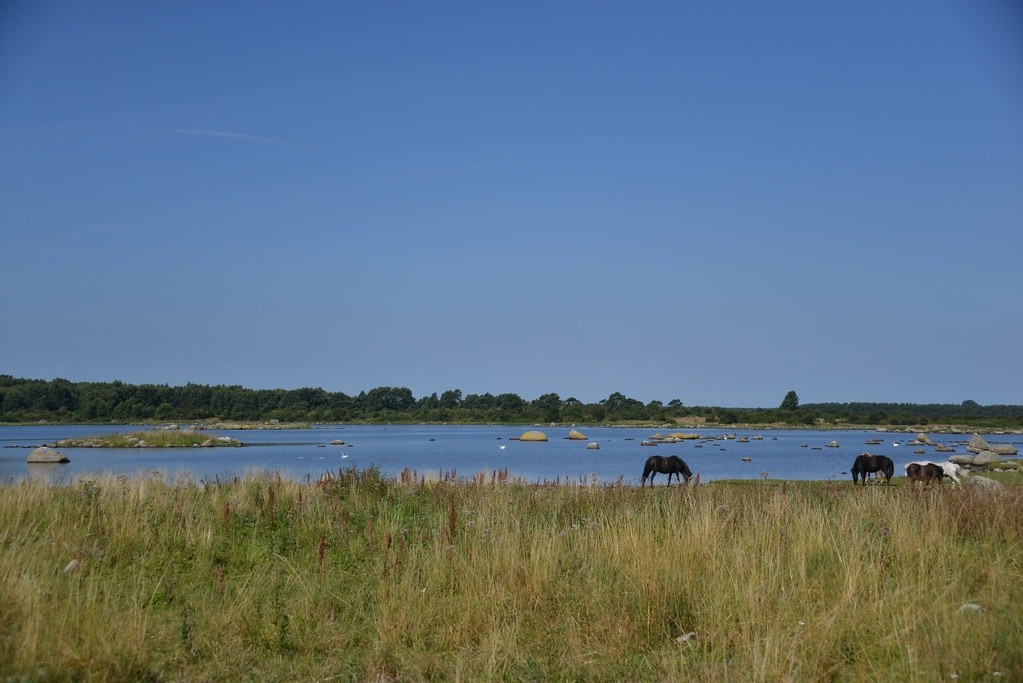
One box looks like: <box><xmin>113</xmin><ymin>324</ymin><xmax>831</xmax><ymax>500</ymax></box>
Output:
<box><xmin>640</xmin><ymin>454</ymin><xmax>692</xmax><ymax>487</ymax></box>
<box><xmin>850</xmin><ymin>452</ymin><xmax>971</xmax><ymax>493</ymax></box>
